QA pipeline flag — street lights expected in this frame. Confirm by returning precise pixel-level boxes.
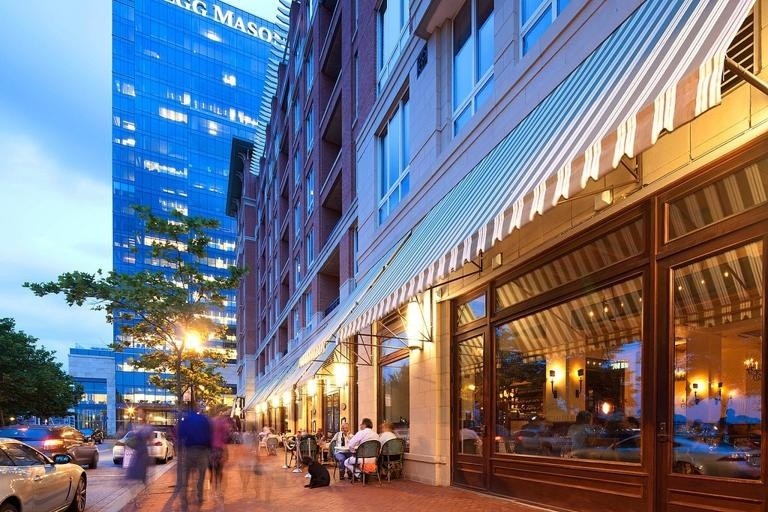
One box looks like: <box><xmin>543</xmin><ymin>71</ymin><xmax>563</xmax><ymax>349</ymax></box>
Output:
<box><xmin>67</xmin><ymin>383</ymin><xmax>79</xmax><ymax>430</ymax></box>
<box><xmin>127</xmin><ymin>405</ymin><xmax>136</xmax><ymax>424</ymax></box>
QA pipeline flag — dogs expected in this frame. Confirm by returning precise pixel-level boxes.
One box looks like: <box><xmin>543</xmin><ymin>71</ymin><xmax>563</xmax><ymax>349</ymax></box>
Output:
<box><xmin>301</xmin><ymin>457</ymin><xmax>330</xmax><ymax>489</ymax></box>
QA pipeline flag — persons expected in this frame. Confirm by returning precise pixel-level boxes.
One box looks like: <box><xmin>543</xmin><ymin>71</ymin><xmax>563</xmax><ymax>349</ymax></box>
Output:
<box><xmin>235</xmin><ymin>422</ymin><xmax>258</xmax><ymax>497</ymax></box>
<box><xmin>119</xmin><ymin>408</ymin><xmax>154</xmax><ymax>508</ymax></box>
<box><xmin>494</xmin><ymin>408</ymin><xmax>760</xmax><ymax>456</ymax></box>
<box><xmin>207</xmin><ymin>410</ymin><xmax>240</xmax><ymax>506</ymax></box>
<box><xmin>172</xmin><ymin>400</ymin><xmax>212</xmax><ymax>511</ymax></box>
<box><xmin>261</xmin><ymin>418</ymin><xmax>400</xmax><ymax>482</ymax></box>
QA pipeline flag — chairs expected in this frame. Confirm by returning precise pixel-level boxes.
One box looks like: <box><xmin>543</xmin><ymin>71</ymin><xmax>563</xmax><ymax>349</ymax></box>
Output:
<box><xmin>257</xmin><ymin>432</ymin><xmax>406</xmax><ymax>487</ymax></box>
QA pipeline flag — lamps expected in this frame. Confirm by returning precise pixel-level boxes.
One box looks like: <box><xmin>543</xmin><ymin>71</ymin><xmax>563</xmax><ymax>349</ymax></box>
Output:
<box><xmin>743</xmin><ymin>357</ymin><xmax>762</xmax><ymax>384</ymax></box>
<box><xmin>549</xmin><ymin>368</ymin><xmax>583</xmax><ymax>398</ymax></box>
<box><xmin>692</xmin><ymin>382</ymin><xmax>723</xmax><ymax>405</ymax></box>
<box><xmin>407</xmin><ymin>300</ymin><xmax>424</xmax><ymax>350</ymax></box>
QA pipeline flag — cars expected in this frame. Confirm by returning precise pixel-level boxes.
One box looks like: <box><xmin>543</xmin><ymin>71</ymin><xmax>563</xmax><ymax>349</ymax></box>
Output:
<box><xmin>389</xmin><ymin>427</ymin><xmax>408</xmax><ymax>451</ymax></box>
<box><xmin>458</xmin><ymin>417</ymin><xmax>762</xmax><ymax>483</ymax></box>
<box><xmin>110</xmin><ymin>428</ymin><xmax>176</xmax><ymax>467</ymax></box>
<box><xmin>0</xmin><ymin>424</ymin><xmax>101</xmax><ymax>471</ymax></box>
<box><xmin>77</xmin><ymin>428</ymin><xmax>105</xmax><ymax>445</ymax></box>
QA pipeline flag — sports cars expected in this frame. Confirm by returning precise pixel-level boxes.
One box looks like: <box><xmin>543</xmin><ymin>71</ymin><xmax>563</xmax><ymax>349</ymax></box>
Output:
<box><xmin>0</xmin><ymin>437</ymin><xmax>88</xmax><ymax>512</ymax></box>
